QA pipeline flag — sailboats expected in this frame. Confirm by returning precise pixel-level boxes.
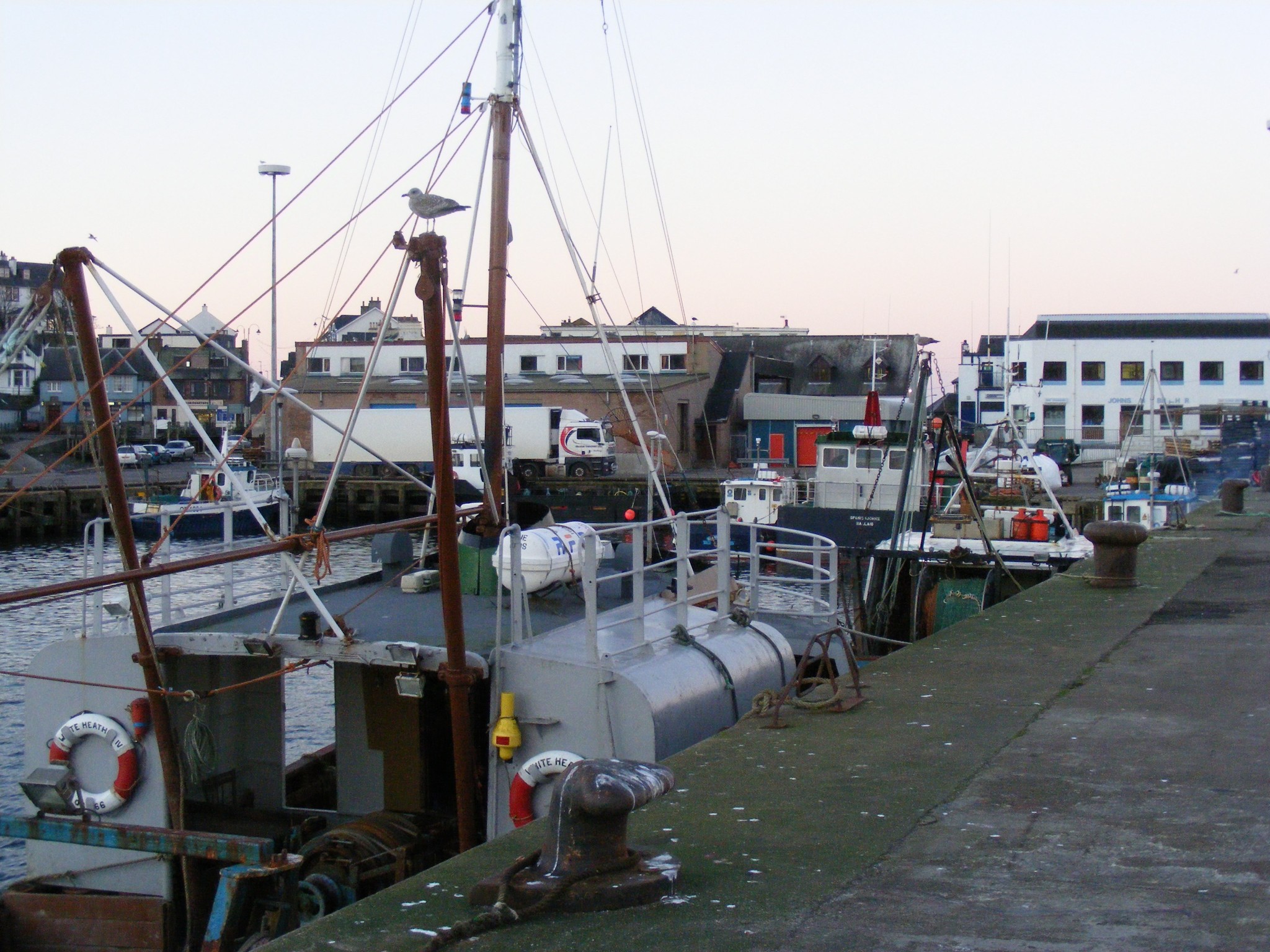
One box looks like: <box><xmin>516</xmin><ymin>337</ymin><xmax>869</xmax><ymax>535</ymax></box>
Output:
<box><xmin>1103</xmin><ymin>349</ymin><xmax>1211</xmax><ymax>535</ymax></box>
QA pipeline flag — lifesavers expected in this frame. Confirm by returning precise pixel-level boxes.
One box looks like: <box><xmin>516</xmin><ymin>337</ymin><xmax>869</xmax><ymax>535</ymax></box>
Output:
<box><xmin>771</xmin><ymin>476</ymin><xmax>785</xmax><ymax>482</ymax></box>
<box><xmin>213</xmin><ymin>485</ymin><xmax>221</xmax><ymax>502</ymax></box>
<box><xmin>48</xmin><ymin>713</ymin><xmax>140</xmax><ymax>815</ymax></box>
<box><xmin>504</xmin><ymin>750</ymin><xmax>585</xmax><ymax>827</ymax></box>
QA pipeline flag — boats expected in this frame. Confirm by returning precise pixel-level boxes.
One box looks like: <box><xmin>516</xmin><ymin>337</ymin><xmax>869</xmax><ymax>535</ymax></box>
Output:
<box><xmin>109</xmin><ymin>418</ymin><xmax>290</xmax><ymax>541</ymax></box>
<box><xmin>0</xmin><ymin>0</ymin><xmax>1093</xmax><ymax>952</ymax></box>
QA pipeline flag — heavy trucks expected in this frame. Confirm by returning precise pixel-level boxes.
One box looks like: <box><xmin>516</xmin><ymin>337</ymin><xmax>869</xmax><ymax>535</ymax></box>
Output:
<box><xmin>313</xmin><ymin>407</ymin><xmax>618</xmax><ymax>482</ymax></box>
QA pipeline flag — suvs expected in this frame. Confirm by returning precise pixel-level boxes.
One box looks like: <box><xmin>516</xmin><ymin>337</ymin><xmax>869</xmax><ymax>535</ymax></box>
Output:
<box><xmin>117</xmin><ymin>445</ymin><xmax>154</xmax><ymax>469</ymax></box>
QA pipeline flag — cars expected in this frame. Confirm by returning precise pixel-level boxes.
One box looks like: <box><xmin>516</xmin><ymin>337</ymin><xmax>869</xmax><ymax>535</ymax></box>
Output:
<box><xmin>228</xmin><ymin>435</ymin><xmax>253</xmax><ymax>452</ymax></box>
<box><xmin>142</xmin><ymin>445</ymin><xmax>172</xmax><ymax>465</ymax></box>
<box><xmin>163</xmin><ymin>440</ymin><xmax>195</xmax><ymax>462</ymax></box>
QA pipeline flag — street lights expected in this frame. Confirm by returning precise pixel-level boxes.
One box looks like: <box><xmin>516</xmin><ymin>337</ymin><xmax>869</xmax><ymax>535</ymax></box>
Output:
<box><xmin>258</xmin><ymin>164</ymin><xmax>291</xmax><ymax>472</ymax></box>
